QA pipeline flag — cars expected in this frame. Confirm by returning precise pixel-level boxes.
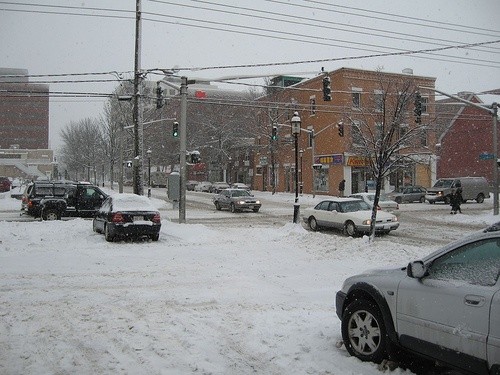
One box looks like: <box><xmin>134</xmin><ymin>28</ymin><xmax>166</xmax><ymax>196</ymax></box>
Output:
<box><xmin>186</xmin><ymin>181</ymin><xmax>249</xmax><ymax>194</ymax></box>
<box><xmin>0</xmin><ymin>176</ymin><xmax>11</xmax><ymax>192</ymax></box>
<box><xmin>92</xmin><ymin>196</ymin><xmax>162</xmax><ymax>243</ymax></box>
<box><xmin>303</xmin><ymin>186</ymin><xmax>427</xmax><ymax>238</ymax></box>
<box><xmin>335</xmin><ymin>223</ymin><xmax>500</xmax><ymax>375</ymax></box>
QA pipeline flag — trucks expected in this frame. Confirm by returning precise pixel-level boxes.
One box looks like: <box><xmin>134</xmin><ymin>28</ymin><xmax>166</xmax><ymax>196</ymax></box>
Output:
<box><xmin>425</xmin><ymin>177</ymin><xmax>490</xmax><ymax>204</ymax></box>
<box><xmin>20</xmin><ymin>180</ymin><xmax>109</xmax><ymax>221</ymax></box>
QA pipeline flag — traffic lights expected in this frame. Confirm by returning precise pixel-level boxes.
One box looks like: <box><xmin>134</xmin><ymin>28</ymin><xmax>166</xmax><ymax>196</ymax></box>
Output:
<box><xmin>338</xmin><ymin>122</ymin><xmax>344</xmax><ymax>136</ymax></box>
<box><xmin>173</xmin><ymin>122</ymin><xmax>179</xmax><ymax>137</ymax></box>
<box><xmin>126</xmin><ymin>162</ymin><xmax>133</xmax><ymax>168</ymax></box>
<box><xmin>191</xmin><ymin>154</ymin><xmax>202</xmax><ymax>164</ymax></box>
<box><xmin>323</xmin><ymin>77</ymin><xmax>332</xmax><ymax>101</ymax></box>
<box><xmin>413</xmin><ymin>94</ymin><xmax>422</xmax><ymax>124</ymax></box>
<box><xmin>272</xmin><ymin>127</ymin><xmax>277</xmax><ymax>140</ymax></box>
<box><xmin>156</xmin><ymin>87</ymin><xmax>164</xmax><ymax>109</ymax></box>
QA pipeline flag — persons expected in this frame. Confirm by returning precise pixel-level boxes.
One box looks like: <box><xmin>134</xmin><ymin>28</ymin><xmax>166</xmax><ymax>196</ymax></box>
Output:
<box><xmin>337</xmin><ymin>180</ymin><xmax>345</xmax><ymax>198</ymax></box>
<box><xmin>449</xmin><ymin>183</ymin><xmax>462</xmax><ymax>214</ymax></box>
<box><xmin>364</xmin><ymin>184</ymin><xmax>368</xmax><ymax>193</ymax></box>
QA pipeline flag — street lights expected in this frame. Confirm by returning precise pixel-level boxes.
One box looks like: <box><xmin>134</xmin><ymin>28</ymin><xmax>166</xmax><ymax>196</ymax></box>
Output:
<box><xmin>299</xmin><ymin>150</ymin><xmax>303</xmax><ymax>194</ymax></box>
<box><xmin>291</xmin><ymin>112</ymin><xmax>302</xmax><ymax>224</ymax></box>
<box><xmin>134</xmin><ymin>69</ymin><xmax>173</xmax><ymax>196</ymax></box>
<box><xmin>229</xmin><ymin>157</ymin><xmax>232</xmax><ymax>182</ymax></box>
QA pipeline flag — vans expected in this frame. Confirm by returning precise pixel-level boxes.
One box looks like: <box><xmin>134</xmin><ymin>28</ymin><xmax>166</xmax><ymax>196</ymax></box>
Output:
<box><xmin>213</xmin><ymin>189</ymin><xmax>261</xmax><ymax>213</ymax></box>
